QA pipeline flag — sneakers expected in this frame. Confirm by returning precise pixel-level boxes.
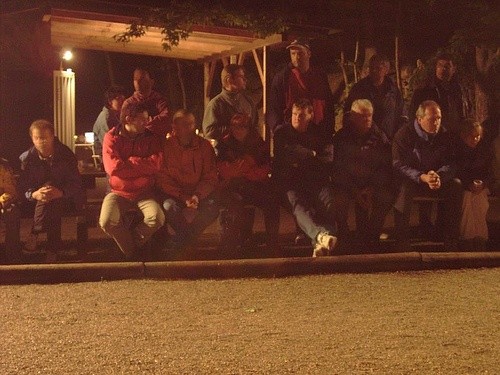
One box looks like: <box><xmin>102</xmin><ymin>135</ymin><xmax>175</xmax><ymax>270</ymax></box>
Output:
<box><xmin>22</xmin><ymin>233</ymin><xmax>38</xmax><ymax>256</ymax></box>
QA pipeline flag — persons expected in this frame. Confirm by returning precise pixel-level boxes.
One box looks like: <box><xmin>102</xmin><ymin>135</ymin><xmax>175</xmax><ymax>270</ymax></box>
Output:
<box><xmin>11</xmin><ymin>119</ymin><xmax>86</xmax><ymax>253</ymax></box>
<box><xmin>156</xmin><ymin>109</ymin><xmax>220</xmax><ymax>249</ymax></box>
<box><xmin>452</xmin><ymin>121</ymin><xmax>492</xmax><ymax>243</ymax></box>
<box><xmin>0</xmin><ymin>163</ymin><xmax>18</xmax><ymax>213</ymax></box>
<box><xmin>329</xmin><ymin>99</ymin><xmax>402</xmax><ymax>242</ymax></box>
<box><xmin>99</xmin><ymin>103</ymin><xmax>166</xmax><ymax>261</ymax></box>
<box><xmin>93</xmin><ymin>35</ymin><xmax>479</xmax><ymax>159</ymax></box>
<box><xmin>273</xmin><ymin>99</ymin><xmax>338</xmax><ymax>257</ymax></box>
<box><xmin>215</xmin><ymin>113</ymin><xmax>280</xmax><ymax>248</ymax></box>
<box><xmin>394</xmin><ymin>100</ymin><xmax>465</xmax><ymax>248</ymax></box>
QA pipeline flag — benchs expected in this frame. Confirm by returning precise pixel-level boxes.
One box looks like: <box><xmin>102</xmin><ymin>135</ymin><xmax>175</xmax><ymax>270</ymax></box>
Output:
<box><xmin>0</xmin><ymin>200</ymin><xmax>441</xmax><ymax>258</ymax></box>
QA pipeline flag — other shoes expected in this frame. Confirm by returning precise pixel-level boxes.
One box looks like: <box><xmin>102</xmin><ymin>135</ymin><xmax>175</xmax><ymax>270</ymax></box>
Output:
<box><xmin>309</xmin><ymin>232</ymin><xmax>337</xmax><ymax>257</ymax></box>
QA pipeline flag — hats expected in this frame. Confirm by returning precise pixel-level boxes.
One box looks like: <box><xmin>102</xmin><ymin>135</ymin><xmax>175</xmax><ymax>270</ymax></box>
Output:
<box><xmin>286</xmin><ymin>37</ymin><xmax>311</xmax><ymax>51</ymax></box>
